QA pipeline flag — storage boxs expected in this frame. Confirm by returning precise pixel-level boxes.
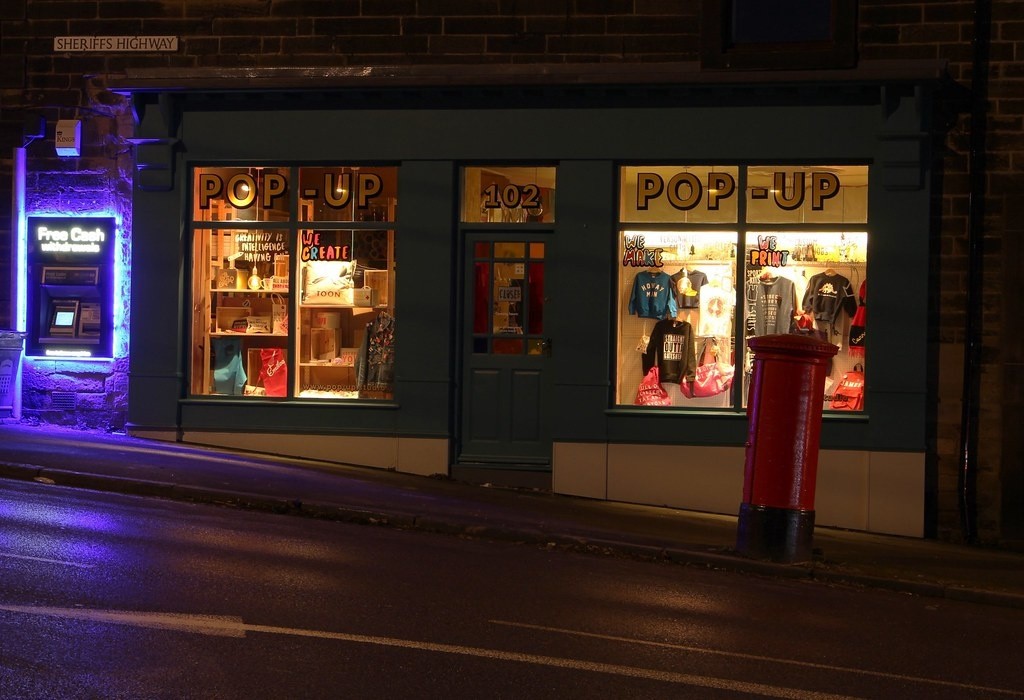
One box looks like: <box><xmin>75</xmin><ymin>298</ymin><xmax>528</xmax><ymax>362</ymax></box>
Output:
<box><xmin>352</xmin><ymin>287</ymin><xmax>378</xmax><ymax>306</ymax></box>
<box><xmin>216</xmin><ymin>268</ymin><xmax>249</xmax><ymax>290</ymax></box>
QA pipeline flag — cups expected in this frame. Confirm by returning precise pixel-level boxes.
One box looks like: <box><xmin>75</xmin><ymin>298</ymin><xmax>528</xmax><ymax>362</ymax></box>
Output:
<box><xmin>259</xmin><ymin>277</ymin><xmax>274</xmax><ymax>289</ymax></box>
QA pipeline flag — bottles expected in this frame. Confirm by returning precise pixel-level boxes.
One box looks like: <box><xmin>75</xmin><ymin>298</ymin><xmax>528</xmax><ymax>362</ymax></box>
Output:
<box><xmin>247</xmin><ymin>266</ymin><xmax>261</xmax><ymax>289</ymax></box>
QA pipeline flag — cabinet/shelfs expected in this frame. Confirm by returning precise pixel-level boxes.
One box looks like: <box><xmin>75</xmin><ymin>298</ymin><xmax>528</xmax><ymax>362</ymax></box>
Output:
<box><xmin>204</xmin><ymin>197</ymin><xmax>289</xmax><ymax>397</ymax></box>
<box><xmin>298</xmin><ymin>197</ymin><xmax>396</xmax><ymax>401</ymax></box>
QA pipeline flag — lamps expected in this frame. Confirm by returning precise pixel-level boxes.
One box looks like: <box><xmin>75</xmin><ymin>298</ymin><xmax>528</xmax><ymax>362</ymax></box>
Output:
<box><xmin>240</xmin><ymin>168</ymin><xmax>254</xmax><ymax>190</ymax></box>
<box><xmin>337</xmin><ymin>167</ymin><xmax>346</xmax><ymax>193</ymax></box>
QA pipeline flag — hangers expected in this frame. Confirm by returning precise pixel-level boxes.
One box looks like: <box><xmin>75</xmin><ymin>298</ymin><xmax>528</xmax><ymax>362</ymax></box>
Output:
<box><xmin>645</xmin><ymin>266</ymin><xmax>662</xmax><ymax>271</ymax></box>
<box><xmin>668</xmin><ymin>316</ymin><xmax>684</xmax><ymax>322</ymax></box>
<box><xmin>758</xmin><ymin>266</ymin><xmax>779</xmax><ymax>276</ymax></box>
<box><xmin>684</xmin><ymin>264</ymin><xmax>693</xmax><ymax>272</ymax></box>
<box><xmin>825</xmin><ymin>268</ymin><xmax>837</xmax><ymax>275</ymax></box>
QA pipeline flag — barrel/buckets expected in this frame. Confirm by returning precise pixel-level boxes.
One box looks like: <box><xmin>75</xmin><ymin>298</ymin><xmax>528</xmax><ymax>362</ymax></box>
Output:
<box><xmin>318</xmin><ymin>312</ymin><xmax>341</xmax><ymax>328</ymax></box>
